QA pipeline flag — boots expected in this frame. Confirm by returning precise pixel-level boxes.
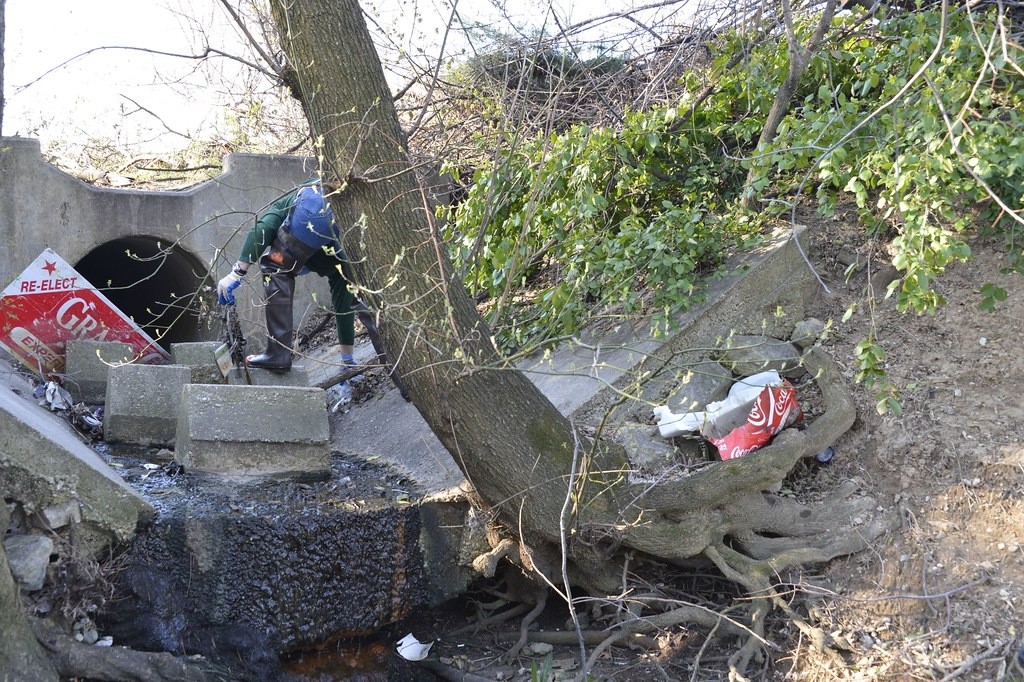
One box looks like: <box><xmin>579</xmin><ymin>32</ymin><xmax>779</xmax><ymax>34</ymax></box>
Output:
<box><xmin>350</xmin><ymin>297</ymin><xmax>412</xmax><ymax>403</ymax></box>
<box><xmin>245</xmin><ymin>225</ymin><xmax>319</xmax><ymax>371</ymax></box>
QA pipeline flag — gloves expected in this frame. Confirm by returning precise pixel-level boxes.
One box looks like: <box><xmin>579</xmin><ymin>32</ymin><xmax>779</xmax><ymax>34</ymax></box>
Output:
<box><xmin>339</xmin><ymin>353</ymin><xmax>363</xmax><ymax>386</ymax></box>
<box><xmin>217</xmin><ymin>273</ymin><xmax>240</xmax><ymax>305</ymax></box>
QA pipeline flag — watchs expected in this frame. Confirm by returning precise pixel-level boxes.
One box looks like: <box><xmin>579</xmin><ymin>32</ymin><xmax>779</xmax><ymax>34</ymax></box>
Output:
<box><xmin>233</xmin><ymin>263</ymin><xmax>246</xmax><ymax>275</ymax></box>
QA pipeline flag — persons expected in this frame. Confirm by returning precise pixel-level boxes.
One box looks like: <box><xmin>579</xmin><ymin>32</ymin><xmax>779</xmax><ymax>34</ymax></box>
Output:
<box><xmin>217</xmin><ymin>177</ymin><xmax>357</xmax><ymax>386</ymax></box>
